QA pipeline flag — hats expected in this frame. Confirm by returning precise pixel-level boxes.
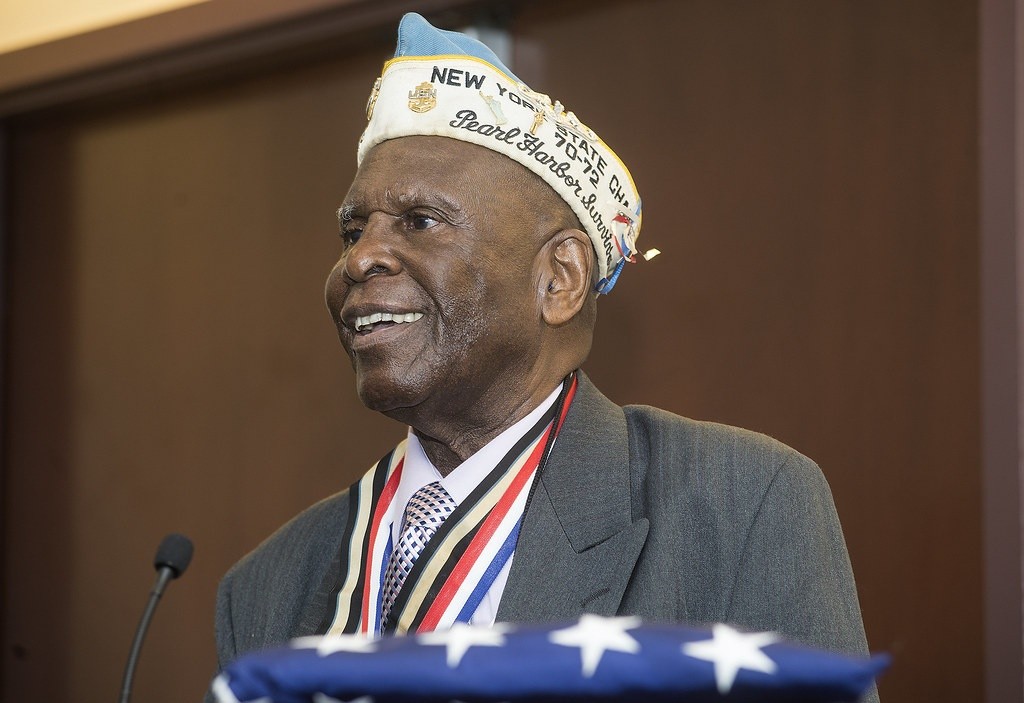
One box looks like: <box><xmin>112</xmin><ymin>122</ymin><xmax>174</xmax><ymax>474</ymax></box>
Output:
<box><xmin>357</xmin><ymin>11</ymin><xmax>642</xmax><ymax>298</ymax></box>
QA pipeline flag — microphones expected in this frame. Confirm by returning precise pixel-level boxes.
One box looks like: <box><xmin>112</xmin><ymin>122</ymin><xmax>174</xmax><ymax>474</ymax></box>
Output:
<box><xmin>117</xmin><ymin>532</ymin><xmax>194</xmax><ymax>702</ymax></box>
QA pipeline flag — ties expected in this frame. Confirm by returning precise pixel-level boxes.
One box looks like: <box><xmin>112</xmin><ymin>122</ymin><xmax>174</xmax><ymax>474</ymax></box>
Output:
<box><xmin>380</xmin><ymin>481</ymin><xmax>456</xmax><ymax>637</ymax></box>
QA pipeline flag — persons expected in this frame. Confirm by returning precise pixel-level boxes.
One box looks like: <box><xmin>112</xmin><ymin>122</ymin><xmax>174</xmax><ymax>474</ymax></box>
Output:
<box><xmin>204</xmin><ymin>12</ymin><xmax>883</xmax><ymax>703</ymax></box>
